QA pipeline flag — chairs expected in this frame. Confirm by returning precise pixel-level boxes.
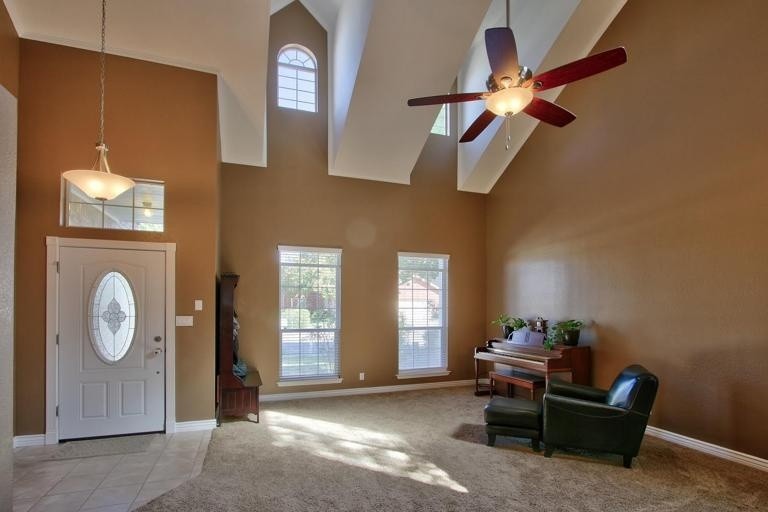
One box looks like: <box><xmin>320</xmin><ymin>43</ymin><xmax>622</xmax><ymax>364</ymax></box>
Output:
<box><xmin>542</xmin><ymin>365</ymin><xmax>659</xmax><ymax>469</ymax></box>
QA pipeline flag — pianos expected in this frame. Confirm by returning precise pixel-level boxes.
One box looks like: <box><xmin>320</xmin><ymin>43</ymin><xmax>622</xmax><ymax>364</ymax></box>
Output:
<box><xmin>472</xmin><ymin>328</ymin><xmax>592</xmax><ymax>398</ymax></box>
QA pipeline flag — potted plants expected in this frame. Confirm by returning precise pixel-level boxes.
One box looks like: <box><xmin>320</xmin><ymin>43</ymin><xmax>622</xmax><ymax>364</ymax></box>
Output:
<box><xmin>543</xmin><ymin>320</ymin><xmax>586</xmax><ymax>352</ymax></box>
<box><xmin>491</xmin><ymin>312</ymin><xmax>527</xmax><ymax>339</ymax></box>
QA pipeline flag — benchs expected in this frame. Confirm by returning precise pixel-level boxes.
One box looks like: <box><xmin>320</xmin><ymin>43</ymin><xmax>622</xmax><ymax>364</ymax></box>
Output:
<box><xmin>488</xmin><ymin>369</ymin><xmax>544</xmax><ymax>400</ymax></box>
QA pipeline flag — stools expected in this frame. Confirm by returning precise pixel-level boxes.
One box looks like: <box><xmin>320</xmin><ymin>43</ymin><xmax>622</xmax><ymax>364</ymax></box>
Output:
<box><xmin>484</xmin><ymin>398</ymin><xmax>542</xmax><ymax>452</ymax></box>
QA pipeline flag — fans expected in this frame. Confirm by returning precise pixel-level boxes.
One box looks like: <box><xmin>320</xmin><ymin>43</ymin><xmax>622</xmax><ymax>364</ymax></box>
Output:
<box><xmin>407</xmin><ymin>1</ymin><xmax>627</xmax><ymax>142</ymax></box>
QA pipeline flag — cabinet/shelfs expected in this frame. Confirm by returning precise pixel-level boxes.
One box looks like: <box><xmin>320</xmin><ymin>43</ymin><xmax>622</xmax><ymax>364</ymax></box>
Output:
<box><xmin>219</xmin><ymin>273</ymin><xmax>263</xmax><ymax>427</ymax></box>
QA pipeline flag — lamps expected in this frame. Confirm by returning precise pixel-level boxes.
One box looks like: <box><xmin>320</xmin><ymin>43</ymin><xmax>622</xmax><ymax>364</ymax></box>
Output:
<box><xmin>485</xmin><ymin>67</ymin><xmax>533</xmax><ymax>118</ymax></box>
<box><xmin>63</xmin><ymin>1</ymin><xmax>135</xmax><ymax>201</ymax></box>
